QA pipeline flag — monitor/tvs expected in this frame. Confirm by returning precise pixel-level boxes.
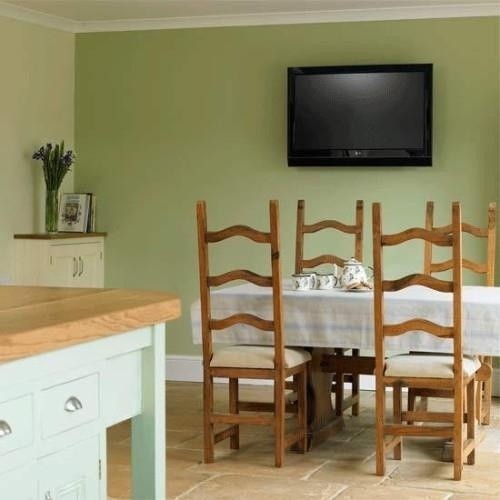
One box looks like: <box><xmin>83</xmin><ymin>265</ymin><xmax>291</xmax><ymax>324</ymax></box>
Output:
<box><xmin>286</xmin><ymin>63</ymin><xmax>433</xmax><ymax>167</ymax></box>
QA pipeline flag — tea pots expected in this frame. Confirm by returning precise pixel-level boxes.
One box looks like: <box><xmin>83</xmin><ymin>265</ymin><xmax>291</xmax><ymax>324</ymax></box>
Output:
<box><xmin>332</xmin><ymin>256</ymin><xmax>375</xmax><ymax>285</ymax></box>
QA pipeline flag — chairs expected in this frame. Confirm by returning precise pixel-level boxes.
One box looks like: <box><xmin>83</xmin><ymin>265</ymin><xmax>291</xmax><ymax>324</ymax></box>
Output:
<box><xmin>282</xmin><ymin>197</ymin><xmax>364</xmax><ymax>419</ymax></box>
<box><xmin>195</xmin><ymin>197</ymin><xmax>308</xmax><ymax>469</ymax></box>
<box><xmin>372</xmin><ymin>200</ymin><xmax>496</xmax><ymax>480</ymax></box>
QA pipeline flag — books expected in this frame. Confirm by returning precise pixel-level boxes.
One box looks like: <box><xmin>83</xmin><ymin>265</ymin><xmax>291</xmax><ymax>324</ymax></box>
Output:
<box><xmin>57</xmin><ymin>192</ymin><xmax>96</xmax><ymax>233</ymax></box>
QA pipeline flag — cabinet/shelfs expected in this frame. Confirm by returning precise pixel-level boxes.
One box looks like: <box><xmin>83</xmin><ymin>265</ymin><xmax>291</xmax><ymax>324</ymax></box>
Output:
<box><xmin>1</xmin><ymin>326</ymin><xmax>172</xmax><ymax>500</ymax></box>
<box><xmin>17</xmin><ymin>237</ymin><xmax>104</xmax><ymax>291</ymax></box>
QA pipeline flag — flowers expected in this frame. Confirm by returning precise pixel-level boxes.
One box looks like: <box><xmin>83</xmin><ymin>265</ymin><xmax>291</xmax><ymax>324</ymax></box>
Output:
<box><xmin>31</xmin><ymin>137</ymin><xmax>79</xmax><ymax>232</ymax></box>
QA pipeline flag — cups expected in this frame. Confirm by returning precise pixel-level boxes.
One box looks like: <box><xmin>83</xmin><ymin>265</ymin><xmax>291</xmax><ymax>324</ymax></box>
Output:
<box><xmin>292</xmin><ymin>271</ymin><xmax>338</xmax><ymax>291</ymax></box>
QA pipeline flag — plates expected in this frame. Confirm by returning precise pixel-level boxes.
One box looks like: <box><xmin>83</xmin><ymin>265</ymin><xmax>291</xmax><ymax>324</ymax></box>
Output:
<box><xmin>337</xmin><ymin>287</ymin><xmax>373</xmax><ymax>293</ymax></box>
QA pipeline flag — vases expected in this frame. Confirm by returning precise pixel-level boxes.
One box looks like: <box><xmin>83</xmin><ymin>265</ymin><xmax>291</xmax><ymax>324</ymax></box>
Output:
<box><xmin>46</xmin><ymin>190</ymin><xmax>58</xmax><ymax>232</ymax></box>
